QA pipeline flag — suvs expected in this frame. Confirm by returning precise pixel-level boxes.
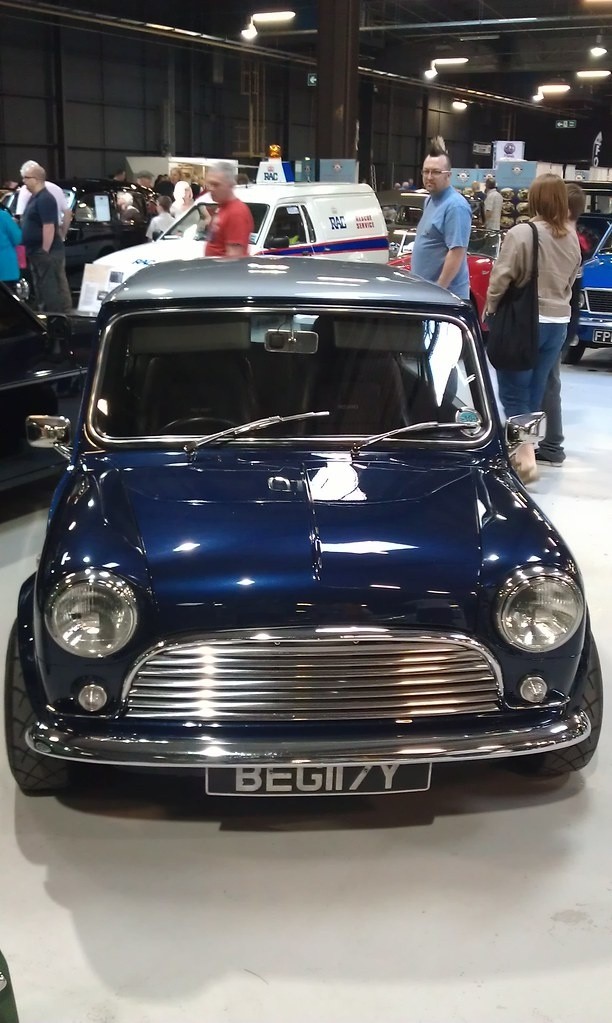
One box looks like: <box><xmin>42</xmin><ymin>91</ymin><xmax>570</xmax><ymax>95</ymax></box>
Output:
<box><xmin>555</xmin><ymin>179</ymin><xmax>612</xmax><ymax>265</ymax></box>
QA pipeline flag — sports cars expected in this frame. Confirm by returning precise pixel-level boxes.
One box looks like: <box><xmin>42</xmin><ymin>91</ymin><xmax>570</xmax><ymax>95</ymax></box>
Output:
<box><xmin>388</xmin><ymin>228</ymin><xmax>507</xmax><ymax>333</ymax></box>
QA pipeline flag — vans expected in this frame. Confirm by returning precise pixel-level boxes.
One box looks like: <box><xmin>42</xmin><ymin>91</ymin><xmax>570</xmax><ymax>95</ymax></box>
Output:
<box><xmin>89</xmin><ymin>162</ymin><xmax>391</xmax><ymax>329</ymax></box>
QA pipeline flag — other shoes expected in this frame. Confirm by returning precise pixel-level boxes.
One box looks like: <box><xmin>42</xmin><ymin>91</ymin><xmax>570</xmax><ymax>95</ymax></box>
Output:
<box><xmin>533</xmin><ymin>448</ymin><xmax>561</xmax><ymax>467</ymax></box>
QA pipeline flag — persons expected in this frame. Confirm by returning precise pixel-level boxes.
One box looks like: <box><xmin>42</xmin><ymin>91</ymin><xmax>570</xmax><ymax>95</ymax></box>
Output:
<box><xmin>412</xmin><ymin>149</ymin><xmax>473</xmax><ymax>302</ymax></box>
<box><xmin>0</xmin><ymin>160</ymin><xmax>72</xmax><ymax>311</ymax></box>
<box><xmin>473</xmin><ymin>178</ymin><xmax>503</xmax><ymax>230</ymax></box>
<box><xmin>533</xmin><ymin>183</ymin><xmax>586</xmax><ymax>467</ymax></box>
<box><xmin>393</xmin><ymin>179</ymin><xmax>415</xmax><ymax>212</ymax></box>
<box><xmin>112</xmin><ymin>167</ymin><xmax>204</xmax><ymax>241</ymax></box>
<box><xmin>204</xmin><ymin>162</ymin><xmax>253</xmax><ymax>257</ymax></box>
<box><xmin>483</xmin><ymin>174</ymin><xmax>582</xmax><ymax>485</ymax></box>
<box><xmin>21</xmin><ymin>165</ymin><xmax>64</xmax><ymax>314</ymax></box>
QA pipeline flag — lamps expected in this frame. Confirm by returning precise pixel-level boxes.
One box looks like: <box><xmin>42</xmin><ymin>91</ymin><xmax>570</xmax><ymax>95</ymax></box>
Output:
<box><xmin>591</xmin><ymin>34</ymin><xmax>606</xmax><ymax>55</ymax></box>
<box><xmin>242</xmin><ymin>18</ymin><xmax>257</xmax><ymax>41</ymax></box>
<box><xmin>425</xmin><ymin>62</ymin><xmax>438</xmax><ymax>78</ymax></box>
<box><xmin>533</xmin><ymin>89</ymin><xmax>544</xmax><ymax>101</ymax></box>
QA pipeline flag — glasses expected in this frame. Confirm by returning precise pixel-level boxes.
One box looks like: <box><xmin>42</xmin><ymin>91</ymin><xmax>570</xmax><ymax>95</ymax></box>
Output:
<box><xmin>21</xmin><ymin>176</ymin><xmax>37</xmax><ymax>181</ymax></box>
<box><xmin>421</xmin><ymin>169</ymin><xmax>451</xmax><ymax>176</ymax></box>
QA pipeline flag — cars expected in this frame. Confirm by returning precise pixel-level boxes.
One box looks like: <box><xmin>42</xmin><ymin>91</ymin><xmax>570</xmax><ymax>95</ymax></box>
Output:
<box><xmin>5</xmin><ymin>177</ymin><xmax>163</xmax><ymax>295</ymax></box>
<box><xmin>2</xmin><ymin>255</ymin><xmax>602</xmax><ymax>798</ymax></box>
<box><xmin>1</xmin><ymin>276</ymin><xmax>96</xmax><ymax>499</ymax></box>
<box><xmin>563</xmin><ymin>224</ymin><xmax>612</xmax><ymax>365</ymax></box>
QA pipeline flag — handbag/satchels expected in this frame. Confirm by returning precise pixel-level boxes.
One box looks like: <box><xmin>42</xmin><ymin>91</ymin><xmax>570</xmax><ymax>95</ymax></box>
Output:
<box><xmin>487</xmin><ymin>221</ymin><xmax>539</xmax><ymax>371</ymax></box>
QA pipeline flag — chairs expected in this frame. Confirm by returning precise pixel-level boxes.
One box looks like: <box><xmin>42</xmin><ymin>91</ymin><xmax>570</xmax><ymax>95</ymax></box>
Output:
<box><xmin>294</xmin><ymin>319</ymin><xmax>414</xmax><ymax>438</ymax></box>
<box><xmin>137</xmin><ymin>349</ymin><xmax>262</xmax><ymax>434</ymax></box>
